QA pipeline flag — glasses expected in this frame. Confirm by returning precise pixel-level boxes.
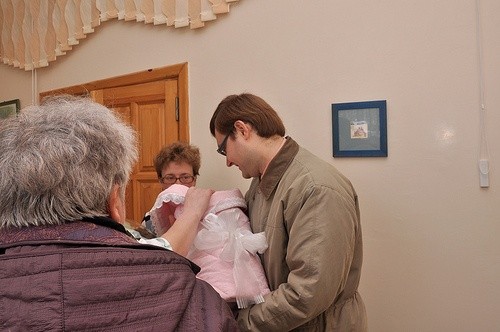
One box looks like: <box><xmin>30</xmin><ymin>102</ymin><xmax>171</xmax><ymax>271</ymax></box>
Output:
<box><xmin>158</xmin><ymin>174</ymin><xmax>196</xmax><ymax>184</ymax></box>
<box><xmin>216</xmin><ymin>128</ymin><xmax>236</xmax><ymax>157</ymax></box>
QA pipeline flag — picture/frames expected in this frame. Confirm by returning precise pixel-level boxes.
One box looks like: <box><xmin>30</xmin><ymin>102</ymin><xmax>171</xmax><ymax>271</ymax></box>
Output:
<box><xmin>0</xmin><ymin>100</ymin><xmax>21</xmax><ymax>118</ymax></box>
<box><xmin>332</xmin><ymin>99</ymin><xmax>387</xmax><ymax>158</ymax></box>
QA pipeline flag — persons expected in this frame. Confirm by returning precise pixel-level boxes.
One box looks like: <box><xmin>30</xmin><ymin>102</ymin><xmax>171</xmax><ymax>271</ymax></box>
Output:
<box><xmin>210</xmin><ymin>93</ymin><xmax>368</xmax><ymax>332</ymax></box>
<box><xmin>118</xmin><ymin>186</ymin><xmax>215</xmax><ymax>258</ymax></box>
<box><xmin>0</xmin><ymin>90</ymin><xmax>238</xmax><ymax>332</ymax></box>
<box><xmin>152</xmin><ymin>142</ymin><xmax>202</xmax><ymax>188</ymax></box>
